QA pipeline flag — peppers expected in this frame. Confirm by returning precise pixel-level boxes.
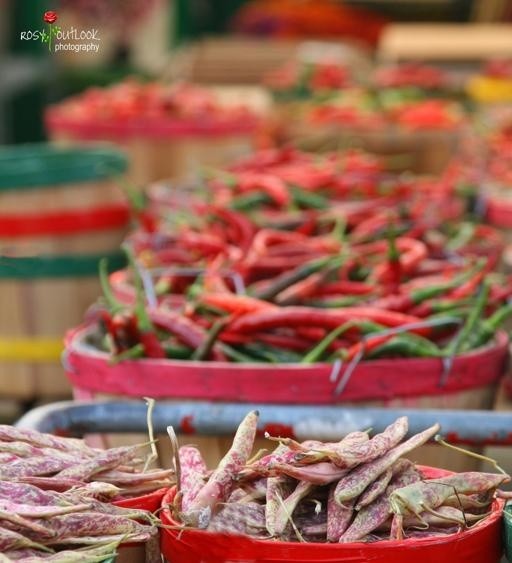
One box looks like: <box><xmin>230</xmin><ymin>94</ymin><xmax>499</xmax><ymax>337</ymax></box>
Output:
<box><xmin>0</xmin><ymin>404</ymin><xmax>511</xmax><ymax>561</ymax></box>
<box><xmin>84</xmin><ymin>145</ymin><xmax>511</xmax><ymax>399</ymax></box>
